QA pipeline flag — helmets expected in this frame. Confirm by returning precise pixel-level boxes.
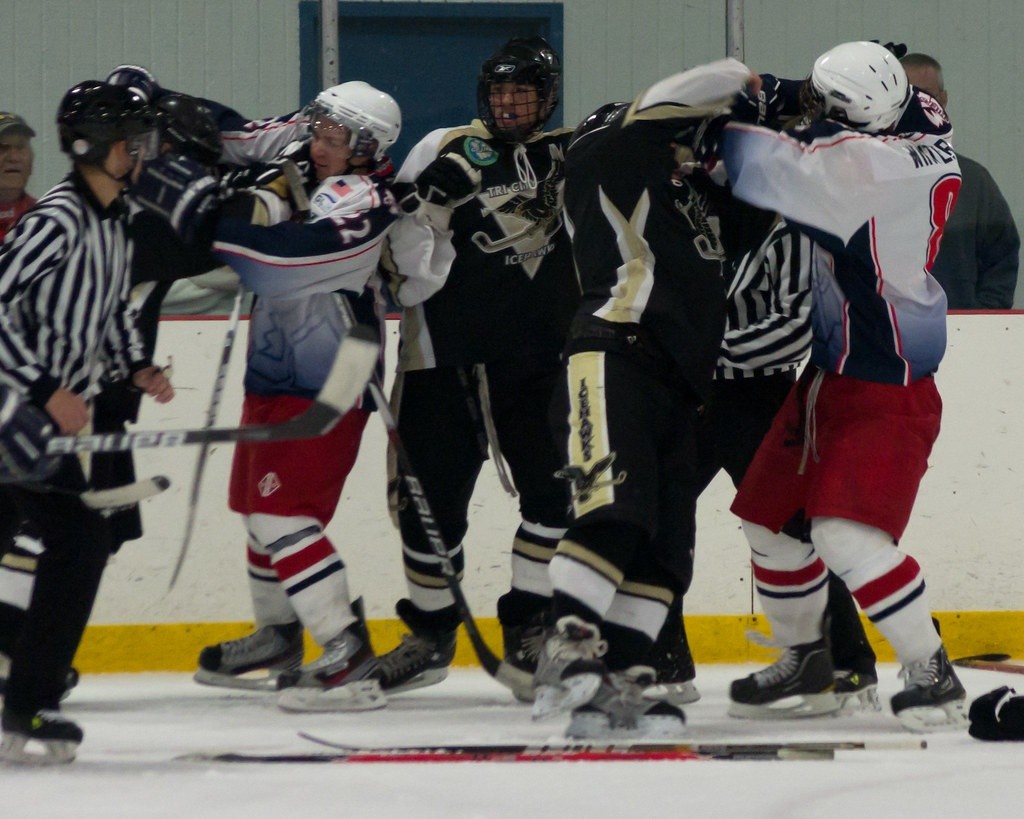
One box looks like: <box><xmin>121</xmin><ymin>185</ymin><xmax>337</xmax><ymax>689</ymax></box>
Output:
<box><xmin>315</xmin><ymin>81</ymin><xmax>401</xmax><ymax>161</ymax></box>
<box><xmin>155</xmin><ymin>93</ymin><xmax>225</xmax><ymax>167</ymax></box>
<box><xmin>54</xmin><ymin>80</ymin><xmax>147</xmax><ymax>165</ymax></box>
<box><xmin>567</xmin><ymin>103</ymin><xmax>627</xmax><ymax>154</ymax></box>
<box><xmin>476</xmin><ymin>36</ymin><xmax>561</xmax><ymax>144</ymax></box>
<box><xmin>799</xmin><ymin>40</ymin><xmax>910</xmax><ymax>135</ymax></box>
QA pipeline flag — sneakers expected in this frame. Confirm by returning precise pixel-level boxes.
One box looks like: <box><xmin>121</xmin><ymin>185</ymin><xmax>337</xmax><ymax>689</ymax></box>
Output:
<box><xmin>193</xmin><ymin>615</ymin><xmax>303</xmax><ymax>690</ymax></box>
<box><xmin>564</xmin><ymin>665</ymin><xmax>686</xmax><ymax>742</ymax></box>
<box><xmin>803</xmin><ymin>644</ymin><xmax>879</xmax><ymax>716</ymax></box>
<box><xmin>532</xmin><ymin>607</ymin><xmax>608</xmax><ymax>718</ymax></box>
<box><xmin>0</xmin><ymin>655</ymin><xmax>78</xmax><ymax>704</ymax></box>
<box><xmin>0</xmin><ymin>712</ymin><xmax>84</xmax><ymax>764</ymax></box>
<box><xmin>278</xmin><ymin>591</ymin><xmax>389</xmax><ymax>712</ymax></box>
<box><xmin>728</xmin><ymin>613</ymin><xmax>839</xmax><ymax>717</ymax></box>
<box><xmin>494</xmin><ymin>595</ymin><xmax>545</xmax><ymax>703</ymax></box>
<box><xmin>643</xmin><ymin>611</ymin><xmax>701</xmax><ymax>706</ymax></box>
<box><xmin>367</xmin><ymin>599</ymin><xmax>469</xmax><ymax>694</ymax></box>
<box><xmin>888</xmin><ymin>617</ymin><xmax>970</xmax><ymax>733</ymax></box>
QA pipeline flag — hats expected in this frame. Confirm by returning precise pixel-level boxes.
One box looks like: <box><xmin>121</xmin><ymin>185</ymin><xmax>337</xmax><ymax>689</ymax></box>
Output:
<box><xmin>0</xmin><ymin>110</ymin><xmax>36</xmax><ymax>138</ymax></box>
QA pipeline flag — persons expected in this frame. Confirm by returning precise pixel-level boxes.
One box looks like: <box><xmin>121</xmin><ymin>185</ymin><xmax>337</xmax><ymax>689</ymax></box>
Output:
<box><xmin>532</xmin><ymin>45</ymin><xmax>966</xmax><ymax>740</ymax></box>
<box><xmin>900</xmin><ymin>53</ymin><xmax>1021</xmax><ymax>310</ymax></box>
<box><xmin>378</xmin><ymin>36</ymin><xmax>580</xmax><ymax>703</ymax></box>
<box><xmin>0</xmin><ymin>64</ymin><xmax>320</xmax><ymax>766</ymax></box>
<box><xmin>135</xmin><ymin>81</ymin><xmax>402</xmax><ymax>713</ymax></box>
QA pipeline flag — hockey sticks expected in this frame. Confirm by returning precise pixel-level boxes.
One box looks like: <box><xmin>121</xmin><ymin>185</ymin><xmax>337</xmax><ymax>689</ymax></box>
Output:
<box><xmin>279</xmin><ymin>157</ymin><xmax>535</xmax><ymax>695</ymax></box>
<box><xmin>79</xmin><ymin>736</ymin><xmax>931</xmax><ymax>767</ymax></box>
<box><xmin>47</xmin><ymin>324</ymin><xmax>386</xmax><ymax>457</ymax></box>
<box><xmin>83</xmin><ymin>475</ymin><xmax>171</xmax><ymax>511</ymax></box>
<box><xmin>166</xmin><ymin>282</ymin><xmax>249</xmax><ymax>596</ymax></box>
<box><xmin>952</xmin><ymin>651</ymin><xmax>1024</xmax><ymax>676</ymax></box>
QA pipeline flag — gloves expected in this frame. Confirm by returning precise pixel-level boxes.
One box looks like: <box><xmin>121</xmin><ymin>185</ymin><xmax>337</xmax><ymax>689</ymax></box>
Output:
<box><xmin>731</xmin><ymin>89</ymin><xmax>783</xmax><ymax>131</ymax></box>
<box><xmin>256</xmin><ymin>134</ymin><xmax>316</xmax><ymax>201</ymax></box>
<box><xmin>565</xmin><ymin>298</ymin><xmax>621</xmax><ymax>358</ymax></box>
<box><xmin>414</xmin><ymin>150</ymin><xmax>482</xmax><ymax>205</ymax></box>
<box><xmin>874</xmin><ymin>37</ymin><xmax>907</xmax><ymax>59</ymax></box>
<box><xmin>690</xmin><ymin>115</ymin><xmax>729</xmax><ymax>161</ymax></box>
